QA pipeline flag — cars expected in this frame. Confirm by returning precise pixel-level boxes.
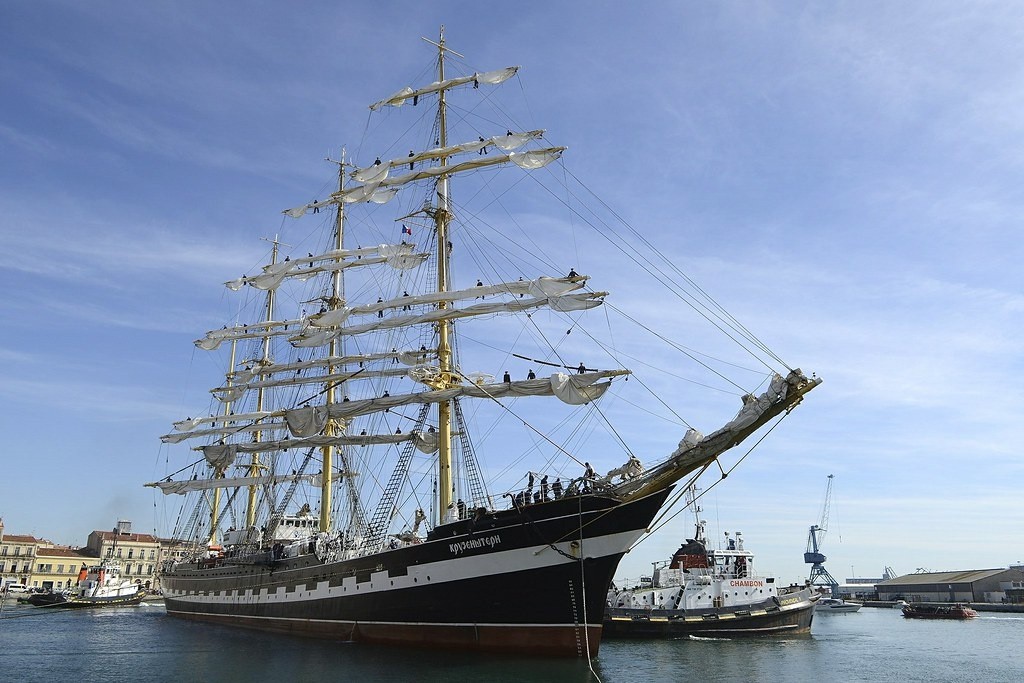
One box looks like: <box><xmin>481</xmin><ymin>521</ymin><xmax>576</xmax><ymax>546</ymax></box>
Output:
<box><xmin>29</xmin><ymin>586</ymin><xmax>78</xmax><ymax>595</ymax></box>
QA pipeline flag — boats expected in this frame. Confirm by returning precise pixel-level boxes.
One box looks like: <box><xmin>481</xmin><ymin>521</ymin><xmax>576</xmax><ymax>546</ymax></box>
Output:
<box><xmin>892</xmin><ymin>599</ymin><xmax>910</xmax><ymax>610</ymax></box>
<box><xmin>601</xmin><ymin>475</ymin><xmax>824</xmax><ymax>641</ymax></box>
<box><xmin>28</xmin><ymin>527</ymin><xmax>149</xmax><ymax>610</ymax></box>
<box><xmin>815</xmin><ymin>598</ymin><xmax>863</xmax><ymax>613</ymax></box>
<box><xmin>901</xmin><ymin>601</ymin><xmax>980</xmax><ymax>620</ymax></box>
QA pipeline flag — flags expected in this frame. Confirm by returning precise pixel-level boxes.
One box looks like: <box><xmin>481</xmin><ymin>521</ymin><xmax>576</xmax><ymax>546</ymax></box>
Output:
<box><xmin>402</xmin><ymin>225</ymin><xmax>411</xmax><ymax>235</ymax></box>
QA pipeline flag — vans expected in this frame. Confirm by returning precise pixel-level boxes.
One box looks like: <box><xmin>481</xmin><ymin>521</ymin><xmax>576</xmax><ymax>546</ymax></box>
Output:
<box><xmin>8</xmin><ymin>583</ymin><xmax>29</xmax><ymax>594</ymax></box>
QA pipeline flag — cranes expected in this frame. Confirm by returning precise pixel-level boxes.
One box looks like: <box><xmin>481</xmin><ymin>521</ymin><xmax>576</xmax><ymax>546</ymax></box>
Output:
<box><xmin>803</xmin><ymin>474</ymin><xmax>840</xmax><ymax>600</ymax></box>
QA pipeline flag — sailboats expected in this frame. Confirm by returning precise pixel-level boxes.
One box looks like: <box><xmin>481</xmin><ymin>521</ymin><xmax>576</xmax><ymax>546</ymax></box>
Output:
<box><xmin>144</xmin><ymin>21</ymin><xmax>824</xmax><ymax>661</ymax></box>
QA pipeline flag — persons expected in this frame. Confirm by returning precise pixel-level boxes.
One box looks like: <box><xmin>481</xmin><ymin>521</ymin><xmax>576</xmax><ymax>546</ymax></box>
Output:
<box><xmin>314</xmin><ymin>199</ymin><xmax>319</xmax><ymax>213</ymax></box>
<box><xmin>402</xmin><ymin>240</ymin><xmax>406</xmax><ymax>245</ymax></box>
<box><xmin>568</xmin><ymin>268</ymin><xmax>579</xmax><ymax>283</ymax></box>
<box><xmin>409</xmin><ymin>150</ymin><xmax>414</xmax><ymax>170</ymax></box>
<box><xmin>507</xmin><ymin>130</ymin><xmax>512</xmax><ymax>136</ymax></box>
<box><xmin>413</xmin><ymin>90</ymin><xmax>418</xmax><ymax>106</ymax></box>
<box><xmin>472</xmin><ymin>72</ymin><xmax>478</xmax><ymax>89</ymax></box>
<box><xmin>169</xmin><ymin>277</ymin><xmax>585</xmax><ymax>482</ymax></box>
<box><xmin>478</xmin><ymin>136</ymin><xmax>487</xmax><ymax>155</ymax></box>
<box><xmin>308</xmin><ymin>253</ymin><xmax>313</xmax><ymax>267</ymax></box>
<box><xmin>243</xmin><ymin>274</ymin><xmax>246</xmax><ymax>285</ymax></box>
<box><xmin>516</xmin><ymin>475</ymin><xmax>562</xmax><ymax>507</ymax></box>
<box><xmin>261</xmin><ymin>526</ymin><xmax>267</xmax><ymax>539</ymax></box>
<box><xmin>585</xmin><ymin>462</ymin><xmax>596</xmax><ymax>489</ymax></box>
<box><xmin>358</xmin><ymin>245</ymin><xmax>361</xmax><ymax>260</ymax></box>
<box><xmin>457</xmin><ymin>499</ymin><xmax>466</xmax><ymax>520</ymax></box>
<box><xmin>285</xmin><ymin>255</ymin><xmax>290</xmax><ymax>261</ymax></box>
<box><xmin>272</xmin><ymin>540</ymin><xmax>286</xmax><ymax>559</ymax></box>
<box><xmin>374</xmin><ymin>157</ymin><xmax>381</xmax><ymax>166</ymax></box>
